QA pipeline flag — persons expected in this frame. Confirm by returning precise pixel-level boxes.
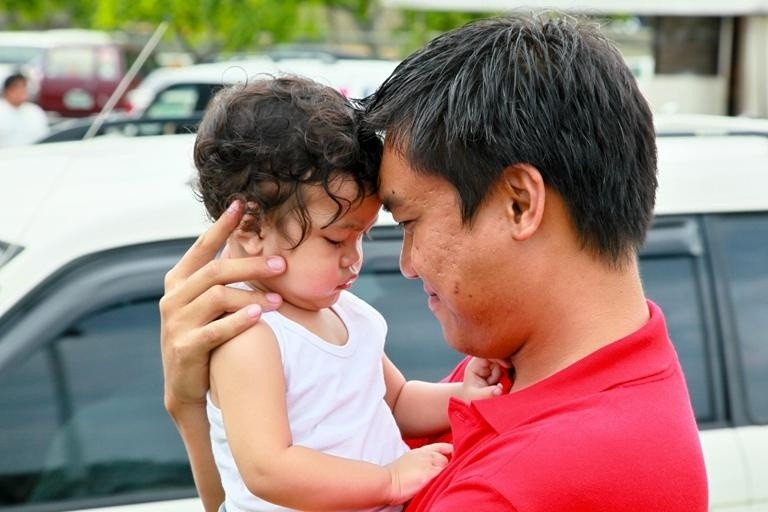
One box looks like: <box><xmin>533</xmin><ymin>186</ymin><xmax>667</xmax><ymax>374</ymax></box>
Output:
<box><xmin>158</xmin><ymin>9</ymin><xmax>708</xmax><ymax>512</ymax></box>
<box><xmin>193</xmin><ymin>65</ymin><xmax>512</xmax><ymax>512</ymax></box>
<box><xmin>0</xmin><ymin>75</ymin><xmax>48</xmax><ymax>147</ymax></box>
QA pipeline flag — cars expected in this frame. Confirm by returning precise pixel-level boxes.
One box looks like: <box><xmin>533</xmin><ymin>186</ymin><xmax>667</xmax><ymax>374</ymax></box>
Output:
<box><xmin>0</xmin><ymin>133</ymin><xmax>767</xmax><ymax>512</ymax></box>
<box><xmin>36</xmin><ymin>40</ymin><xmax>167</xmax><ymax>116</ymax></box>
